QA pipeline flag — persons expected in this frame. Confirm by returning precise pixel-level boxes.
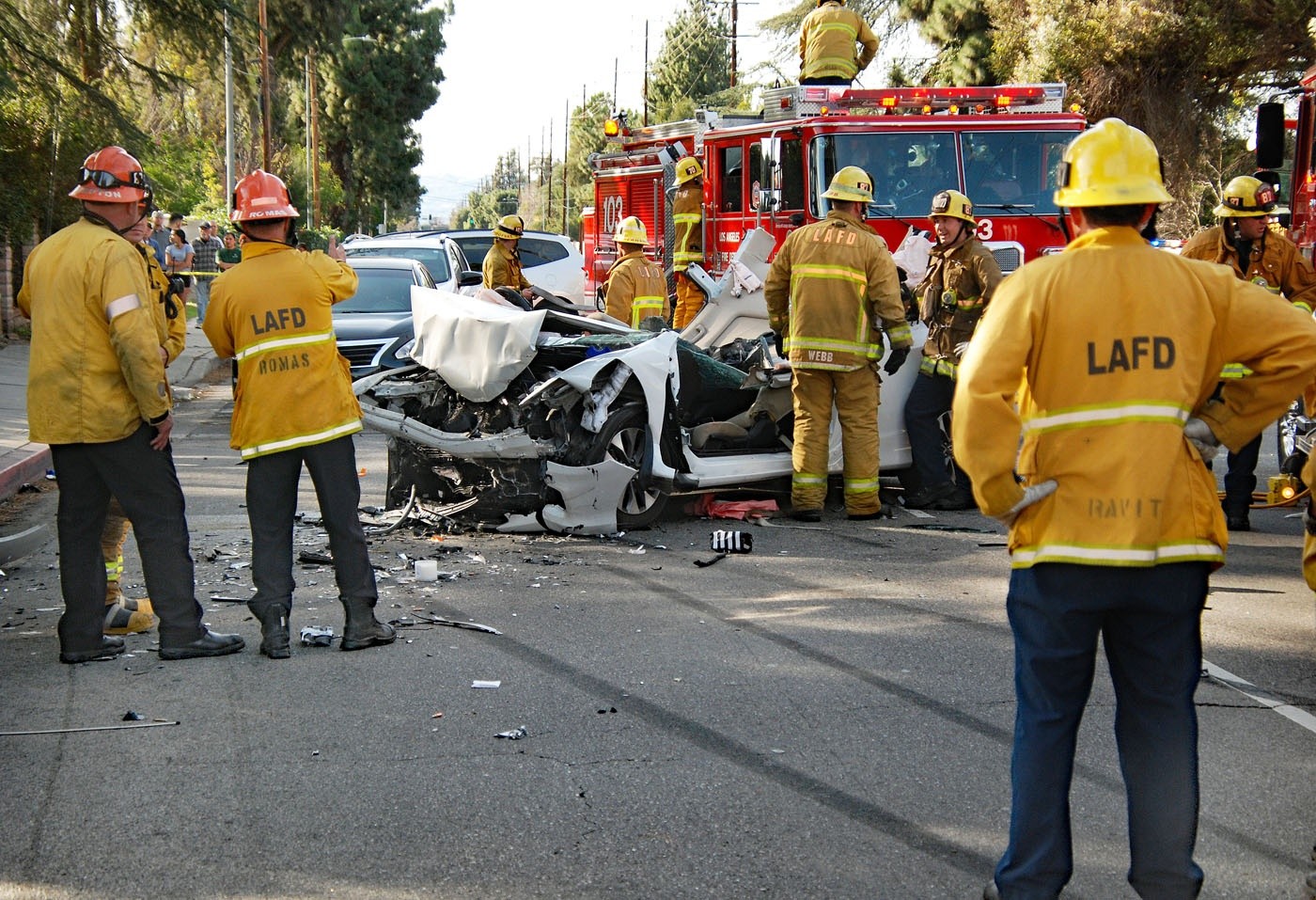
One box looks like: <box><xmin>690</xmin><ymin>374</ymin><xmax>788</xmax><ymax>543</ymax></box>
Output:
<box><xmin>165</xmin><ymin>228</ymin><xmax>195</xmax><ymax>307</ymax></box>
<box><xmin>214</xmin><ymin>232</ymin><xmax>242</xmax><ymax>276</ymax></box>
<box><xmin>163</xmin><ymin>213</ymin><xmax>183</xmax><ymax>233</ymax></box>
<box><xmin>953</xmin><ymin>118</ymin><xmax>1315</xmax><ymax>900</ymax></box>
<box><xmin>191</xmin><ymin>222</ymin><xmax>220</xmax><ymax>329</ymax></box>
<box><xmin>150</xmin><ymin>210</ymin><xmax>172</xmax><ymax>269</ymax></box>
<box><xmin>481</xmin><ymin>214</ymin><xmax>539</xmax><ymax>300</ymax></box>
<box><xmin>297</xmin><ymin>243</ymin><xmax>307</xmax><ymax>253</ymax></box>
<box><xmin>671</xmin><ymin>157</ymin><xmax>705</xmax><ymax>332</ymax></box>
<box><xmin>202</xmin><ymin>169</ymin><xmax>396</xmax><ymax>660</ymax></box>
<box><xmin>584</xmin><ymin>217</ymin><xmax>670</xmax><ymax>335</ymax></box>
<box><xmin>799</xmin><ymin>0</ymin><xmax>879</xmax><ymax>91</ymax></box>
<box><xmin>141</xmin><ymin>223</ymin><xmax>164</xmax><ymax>268</ymax></box>
<box><xmin>902</xmin><ymin>189</ymin><xmax>1001</xmax><ymax>511</ymax></box>
<box><xmin>207</xmin><ymin>220</ymin><xmax>225</xmax><ymax>250</ymax></box>
<box><xmin>762</xmin><ymin>166</ymin><xmax>914</xmax><ymax>520</ymax></box>
<box><xmin>95</xmin><ymin>189</ymin><xmax>185</xmax><ymax>634</ymax></box>
<box><xmin>16</xmin><ymin>145</ymin><xmax>246</xmax><ymax>663</ymax></box>
<box><xmin>1180</xmin><ymin>175</ymin><xmax>1316</xmax><ymax>528</ymax></box>
<box><xmin>1299</xmin><ymin>439</ymin><xmax>1315</xmax><ymax>594</ymax></box>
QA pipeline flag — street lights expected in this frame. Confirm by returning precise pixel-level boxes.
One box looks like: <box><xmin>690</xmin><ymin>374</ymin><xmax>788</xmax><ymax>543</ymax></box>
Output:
<box><xmin>305</xmin><ymin>34</ymin><xmax>376</xmax><ymax>231</ymax></box>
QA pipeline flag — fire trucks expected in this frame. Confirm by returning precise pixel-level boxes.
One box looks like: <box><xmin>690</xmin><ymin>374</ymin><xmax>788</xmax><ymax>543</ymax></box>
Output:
<box><xmin>582</xmin><ymin>84</ymin><xmax>1093</xmax><ymax>313</ymax></box>
<box><xmin>1254</xmin><ymin>58</ymin><xmax>1316</xmax><ymax>493</ymax></box>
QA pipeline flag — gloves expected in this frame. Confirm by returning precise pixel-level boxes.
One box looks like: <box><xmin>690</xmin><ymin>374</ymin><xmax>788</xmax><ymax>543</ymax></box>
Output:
<box><xmin>883</xmin><ymin>348</ymin><xmax>909</xmax><ymax>375</ymax></box>
<box><xmin>772</xmin><ymin>335</ymin><xmax>789</xmax><ymax>360</ymax></box>
<box><xmin>1184</xmin><ymin>416</ymin><xmax>1220</xmax><ymax>464</ymax></box>
<box><xmin>1001</xmin><ymin>481</ymin><xmax>1058</xmax><ymax>528</ymax></box>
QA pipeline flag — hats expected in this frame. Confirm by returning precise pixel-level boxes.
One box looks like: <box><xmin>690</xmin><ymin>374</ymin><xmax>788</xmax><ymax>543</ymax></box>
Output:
<box><xmin>198</xmin><ymin>222</ymin><xmax>210</xmax><ymax>229</ymax></box>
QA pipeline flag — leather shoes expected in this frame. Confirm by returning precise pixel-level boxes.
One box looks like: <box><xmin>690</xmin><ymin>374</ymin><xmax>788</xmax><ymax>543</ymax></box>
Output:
<box><xmin>59</xmin><ymin>636</ymin><xmax>125</xmax><ymax>663</ymax></box>
<box><xmin>158</xmin><ymin>631</ymin><xmax>245</xmax><ymax>660</ymax></box>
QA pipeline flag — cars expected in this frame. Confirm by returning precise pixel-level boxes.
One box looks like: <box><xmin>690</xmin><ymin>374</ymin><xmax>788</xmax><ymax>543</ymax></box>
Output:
<box><xmin>330</xmin><ymin>259</ymin><xmax>439</xmax><ymax>396</ymax></box>
<box><xmin>351</xmin><ymin>227</ymin><xmax>953</xmax><ymax>538</ymax></box>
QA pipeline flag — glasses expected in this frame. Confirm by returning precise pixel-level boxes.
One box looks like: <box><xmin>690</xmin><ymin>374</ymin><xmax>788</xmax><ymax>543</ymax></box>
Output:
<box><xmin>77</xmin><ymin>166</ymin><xmax>120</xmax><ymax>187</ymax></box>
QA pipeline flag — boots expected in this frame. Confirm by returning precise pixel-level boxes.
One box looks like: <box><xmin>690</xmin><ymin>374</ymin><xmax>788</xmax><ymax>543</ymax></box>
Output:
<box><xmin>259</xmin><ymin>603</ymin><xmax>291</xmax><ymax>658</ymax></box>
<box><xmin>340</xmin><ymin>596</ymin><xmax>398</xmax><ymax>649</ymax></box>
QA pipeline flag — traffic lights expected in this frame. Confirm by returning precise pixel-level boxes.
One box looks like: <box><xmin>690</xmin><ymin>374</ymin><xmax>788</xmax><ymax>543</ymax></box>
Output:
<box><xmin>470</xmin><ymin>217</ymin><xmax>474</xmax><ymax>227</ymax></box>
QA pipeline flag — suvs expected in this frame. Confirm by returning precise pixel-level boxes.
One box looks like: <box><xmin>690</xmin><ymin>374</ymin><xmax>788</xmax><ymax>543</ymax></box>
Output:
<box><xmin>335</xmin><ymin>233</ymin><xmax>483</xmax><ymax>297</ymax></box>
<box><xmin>373</xmin><ymin>229</ymin><xmax>585</xmax><ymax>336</ymax></box>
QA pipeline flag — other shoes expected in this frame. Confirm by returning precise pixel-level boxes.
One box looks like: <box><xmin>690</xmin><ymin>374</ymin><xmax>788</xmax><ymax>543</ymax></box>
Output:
<box><xmin>984</xmin><ymin>879</ymin><xmax>1002</xmax><ymax>900</ymax></box>
<box><xmin>120</xmin><ymin>596</ymin><xmax>154</xmax><ymax>615</ymax></box>
<box><xmin>103</xmin><ymin>604</ymin><xmax>154</xmax><ymax>634</ymax></box>
<box><xmin>1227</xmin><ymin>516</ymin><xmax>1248</xmax><ymax>529</ymax></box>
<box><xmin>905</xmin><ymin>480</ymin><xmax>957</xmax><ymax>508</ymax></box>
<box><xmin>793</xmin><ymin>510</ymin><xmax>821</xmax><ymax>521</ymax></box>
<box><xmin>850</xmin><ymin>506</ymin><xmax>893</xmax><ymax>519</ymax></box>
<box><xmin>936</xmin><ymin>484</ymin><xmax>972</xmax><ymax>510</ymax></box>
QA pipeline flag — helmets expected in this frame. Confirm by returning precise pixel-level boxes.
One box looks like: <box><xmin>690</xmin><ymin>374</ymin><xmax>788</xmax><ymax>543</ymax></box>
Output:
<box><xmin>1053</xmin><ymin>118</ymin><xmax>1177</xmax><ymax>207</ymax></box>
<box><xmin>1212</xmin><ymin>176</ymin><xmax>1279</xmax><ymax>218</ymax></box>
<box><xmin>671</xmin><ymin>156</ymin><xmax>702</xmax><ymax>186</ymax></box>
<box><xmin>231</xmin><ymin>169</ymin><xmax>300</xmax><ymax>221</ymax></box>
<box><xmin>611</xmin><ymin>216</ymin><xmax>650</xmax><ymax>245</ymax></box>
<box><xmin>68</xmin><ymin>146</ymin><xmax>145</xmax><ymax>202</ymax></box>
<box><xmin>928</xmin><ymin>189</ymin><xmax>977</xmax><ymax>228</ymax></box>
<box><xmin>491</xmin><ymin>215</ymin><xmax>522</xmax><ymax>239</ymax></box>
<box><xmin>820</xmin><ymin>167</ymin><xmax>875</xmax><ymax>203</ymax></box>
